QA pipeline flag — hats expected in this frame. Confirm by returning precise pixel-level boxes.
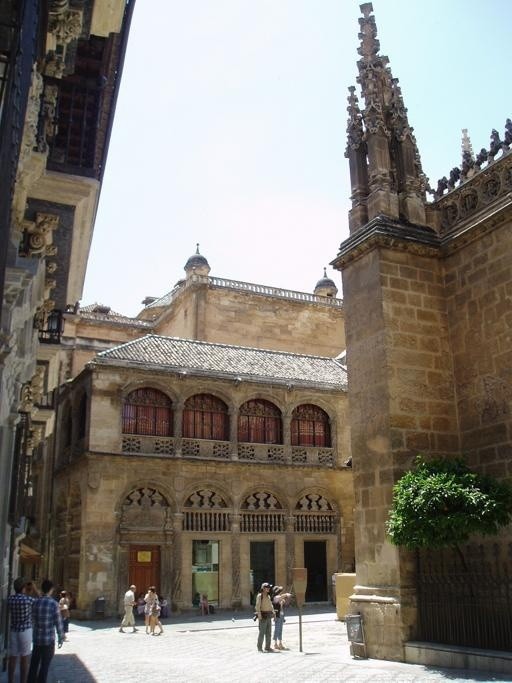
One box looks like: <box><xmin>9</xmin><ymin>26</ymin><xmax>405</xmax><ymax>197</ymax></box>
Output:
<box><xmin>261</xmin><ymin>582</ymin><xmax>274</xmax><ymax>590</ymax></box>
<box><xmin>271</xmin><ymin>585</ymin><xmax>283</xmax><ymax>595</ymax></box>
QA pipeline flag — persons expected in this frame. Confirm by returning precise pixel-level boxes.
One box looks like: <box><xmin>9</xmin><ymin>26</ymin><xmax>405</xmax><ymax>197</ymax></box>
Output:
<box><xmin>59</xmin><ymin>590</ymin><xmax>71</xmax><ymax>633</ymax></box>
<box><xmin>119</xmin><ymin>584</ymin><xmax>139</xmax><ymax>633</ymax></box>
<box><xmin>255</xmin><ymin>582</ymin><xmax>277</xmax><ymax>652</ymax></box>
<box><xmin>137</xmin><ymin>585</ymin><xmax>168</xmax><ymax>635</ymax></box>
<box><xmin>26</xmin><ymin>579</ymin><xmax>65</xmax><ymax>683</ymax></box>
<box><xmin>271</xmin><ymin>586</ymin><xmax>292</xmax><ymax>650</ymax></box>
<box><xmin>198</xmin><ymin>592</ymin><xmax>209</xmax><ymax>616</ymax></box>
<box><xmin>6</xmin><ymin>576</ymin><xmax>34</xmax><ymax>683</ymax></box>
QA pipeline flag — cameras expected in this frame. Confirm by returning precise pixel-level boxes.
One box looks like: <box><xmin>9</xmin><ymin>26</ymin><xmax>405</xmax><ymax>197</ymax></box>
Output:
<box><xmin>27</xmin><ymin>582</ymin><xmax>32</xmax><ymax>586</ymax></box>
<box><xmin>252</xmin><ymin>612</ymin><xmax>257</xmax><ymax>621</ymax></box>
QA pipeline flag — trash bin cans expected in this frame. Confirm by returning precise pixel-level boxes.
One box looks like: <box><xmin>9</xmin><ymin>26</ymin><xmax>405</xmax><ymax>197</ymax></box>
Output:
<box><xmin>344</xmin><ymin>614</ymin><xmax>367</xmax><ymax>659</ymax></box>
<box><xmin>94</xmin><ymin>596</ymin><xmax>106</xmax><ymax>619</ymax></box>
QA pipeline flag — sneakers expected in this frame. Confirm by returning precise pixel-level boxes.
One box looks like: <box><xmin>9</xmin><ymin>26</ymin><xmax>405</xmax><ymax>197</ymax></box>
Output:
<box><xmin>119</xmin><ymin>627</ymin><xmax>139</xmax><ymax>635</ymax></box>
<box><xmin>252</xmin><ymin>645</ymin><xmax>284</xmax><ymax>653</ymax></box>
